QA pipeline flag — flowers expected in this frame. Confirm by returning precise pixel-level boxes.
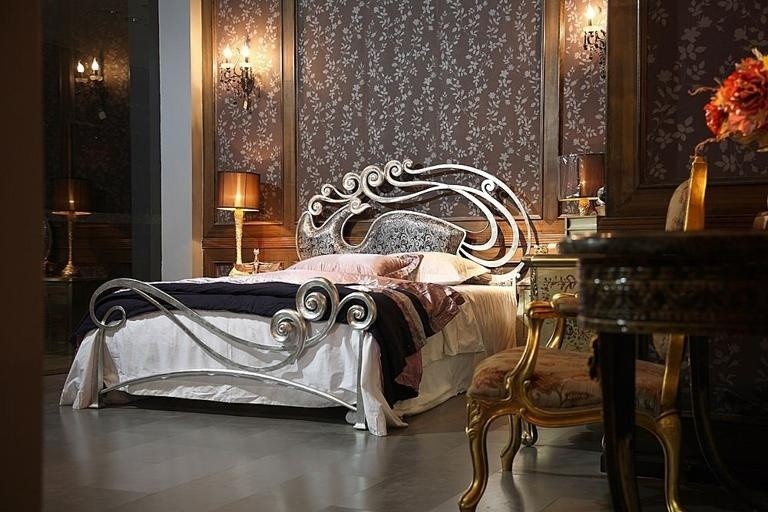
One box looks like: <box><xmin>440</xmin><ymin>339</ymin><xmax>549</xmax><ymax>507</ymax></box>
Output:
<box><xmin>688</xmin><ymin>48</ymin><xmax>768</xmax><ymax>154</ymax></box>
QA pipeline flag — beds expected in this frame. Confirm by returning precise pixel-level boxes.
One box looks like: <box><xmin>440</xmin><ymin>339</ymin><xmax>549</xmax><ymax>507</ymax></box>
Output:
<box><xmin>60</xmin><ymin>159</ymin><xmax>533</xmax><ymax>437</ymax></box>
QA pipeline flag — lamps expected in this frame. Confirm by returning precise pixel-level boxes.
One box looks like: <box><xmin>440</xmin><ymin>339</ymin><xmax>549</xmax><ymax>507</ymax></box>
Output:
<box><xmin>220</xmin><ymin>35</ymin><xmax>277</xmax><ymax>114</ymax></box>
<box><xmin>76</xmin><ymin>56</ymin><xmax>104</xmax><ymax>118</ymax></box>
<box><xmin>52</xmin><ymin>197</ymin><xmax>92</xmax><ymax>275</ymax></box>
<box><xmin>562</xmin><ymin>153</ymin><xmax>605</xmax><ymax>216</ymax></box>
<box><xmin>581</xmin><ymin>10</ymin><xmax>606</xmax><ymax>77</ymax></box>
<box><xmin>215</xmin><ymin>171</ymin><xmax>259</xmax><ymax>273</ymax></box>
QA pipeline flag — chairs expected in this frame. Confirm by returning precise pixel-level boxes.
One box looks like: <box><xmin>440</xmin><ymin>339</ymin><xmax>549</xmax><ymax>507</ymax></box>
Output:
<box><xmin>456</xmin><ymin>159</ymin><xmax>707</xmax><ymax>511</ymax></box>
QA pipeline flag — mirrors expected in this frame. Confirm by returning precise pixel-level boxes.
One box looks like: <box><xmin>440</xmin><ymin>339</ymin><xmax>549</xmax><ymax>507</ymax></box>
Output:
<box><xmin>40</xmin><ymin>0</ymin><xmax>159</xmax><ymax>376</ymax></box>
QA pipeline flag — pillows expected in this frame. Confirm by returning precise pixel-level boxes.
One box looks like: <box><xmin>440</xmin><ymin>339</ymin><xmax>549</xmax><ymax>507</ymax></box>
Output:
<box><xmin>393</xmin><ymin>250</ymin><xmax>490</xmax><ymax>285</ymax></box>
<box><xmin>286</xmin><ymin>254</ymin><xmax>423</xmax><ymax>284</ymax></box>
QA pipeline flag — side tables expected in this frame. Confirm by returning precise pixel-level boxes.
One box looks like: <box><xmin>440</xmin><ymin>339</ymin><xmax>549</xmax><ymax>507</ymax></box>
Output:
<box><xmin>556</xmin><ymin>230</ymin><xmax>767</xmax><ymax>510</ymax></box>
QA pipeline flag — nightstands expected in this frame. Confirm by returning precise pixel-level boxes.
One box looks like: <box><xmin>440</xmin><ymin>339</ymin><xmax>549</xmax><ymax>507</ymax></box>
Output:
<box><xmin>44</xmin><ymin>273</ymin><xmax>107</xmax><ymax>353</ymax></box>
<box><xmin>525</xmin><ymin>254</ymin><xmax>598</xmax><ymax>354</ymax></box>
